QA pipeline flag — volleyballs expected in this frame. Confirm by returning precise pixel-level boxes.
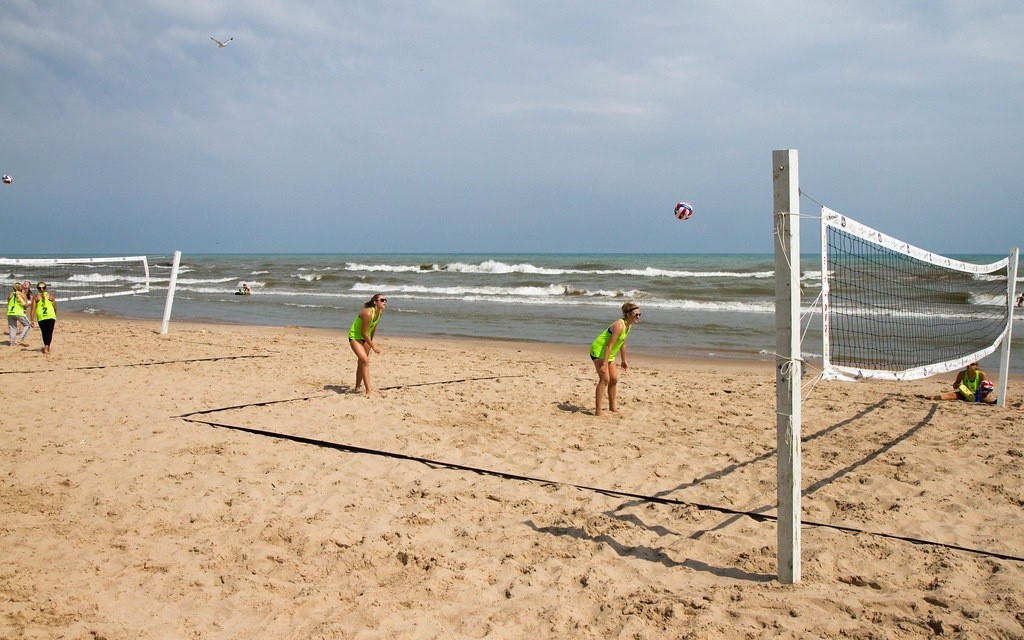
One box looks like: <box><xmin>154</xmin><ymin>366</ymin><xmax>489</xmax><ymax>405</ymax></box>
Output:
<box><xmin>674</xmin><ymin>201</ymin><xmax>693</xmax><ymax>221</ymax></box>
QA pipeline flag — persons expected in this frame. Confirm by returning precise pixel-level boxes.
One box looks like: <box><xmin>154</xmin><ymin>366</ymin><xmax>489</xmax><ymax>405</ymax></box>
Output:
<box><xmin>930</xmin><ymin>360</ymin><xmax>997</xmax><ymax>405</ymax></box>
<box><xmin>348</xmin><ymin>293</ymin><xmax>388</xmax><ymax>394</ymax></box>
<box><xmin>7</xmin><ymin>281</ymin><xmax>57</xmax><ymax>355</ymax></box>
<box><xmin>589</xmin><ymin>302</ymin><xmax>641</xmax><ymax>417</ymax></box>
<box><xmin>239</xmin><ymin>284</ymin><xmax>251</xmax><ymax>295</ymax></box>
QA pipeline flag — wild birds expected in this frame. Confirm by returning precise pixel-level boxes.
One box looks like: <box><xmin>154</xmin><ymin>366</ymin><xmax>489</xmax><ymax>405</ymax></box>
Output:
<box><xmin>211</xmin><ymin>38</ymin><xmax>233</xmax><ymax>48</ymax></box>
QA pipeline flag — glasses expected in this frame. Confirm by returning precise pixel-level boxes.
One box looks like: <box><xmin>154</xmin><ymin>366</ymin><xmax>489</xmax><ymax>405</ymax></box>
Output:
<box><xmin>631</xmin><ymin>313</ymin><xmax>641</xmax><ymax>317</ymax></box>
<box><xmin>376</xmin><ymin>299</ymin><xmax>387</xmax><ymax>302</ymax></box>
<box><xmin>970</xmin><ymin>363</ymin><xmax>976</xmax><ymax>366</ymax></box>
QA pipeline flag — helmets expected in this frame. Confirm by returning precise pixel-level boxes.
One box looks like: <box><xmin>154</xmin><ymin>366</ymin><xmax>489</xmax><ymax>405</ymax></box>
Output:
<box><xmin>980</xmin><ymin>380</ymin><xmax>994</xmax><ymax>393</ymax></box>
